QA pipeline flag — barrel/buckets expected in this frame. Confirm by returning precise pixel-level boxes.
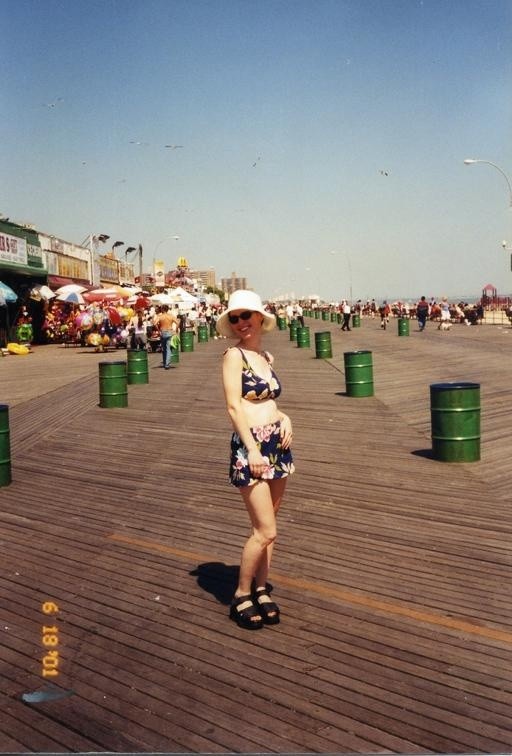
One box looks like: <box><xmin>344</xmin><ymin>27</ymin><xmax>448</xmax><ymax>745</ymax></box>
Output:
<box><xmin>289</xmin><ymin>323</ymin><xmax>302</xmax><ymax>341</ymax></box>
<box><xmin>431</xmin><ymin>382</ymin><xmax>482</xmax><ymax>462</ymax></box>
<box><xmin>126</xmin><ymin>348</ymin><xmax>148</xmax><ymax>385</ymax></box>
<box><xmin>398</xmin><ymin>319</ymin><xmax>410</xmax><ymax>336</ymax></box>
<box><xmin>181</xmin><ymin>331</ymin><xmax>194</xmax><ymax>352</ymax></box>
<box><xmin>171</xmin><ymin>337</ymin><xmax>179</xmax><ymax>363</ymax></box>
<box><xmin>345</xmin><ymin>350</ymin><xmax>373</xmax><ymax>396</ymax></box>
<box><xmin>353</xmin><ymin>314</ymin><xmax>361</xmax><ymax>328</ymax></box>
<box><xmin>1</xmin><ymin>406</ymin><xmax>12</xmax><ymax>483</ymax></box>
<box><xmin>198</xmin><ymin>326</ymin><xmax>207</xmax><ymax>342</ymax></box>
<box><xmin>297</xmin><ymin>327</ymin><xmax>310</xmax><ymax>348</ymax></box>
<box><xmin>99</xmin><ymin>361</ymin><xmax>129</xmax><ymax>407</ymax></box>
<box><xmin>315</xmin><ymin>331</ymin><xmax>332</xmax><ymax>358</ymax></box>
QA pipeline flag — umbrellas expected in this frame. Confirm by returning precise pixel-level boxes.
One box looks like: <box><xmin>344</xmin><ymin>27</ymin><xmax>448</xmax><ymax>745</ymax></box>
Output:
<box><xmin>0</xmin><ymin>280</ymin><xmax>199</xmax><ymax>314</ymax></box>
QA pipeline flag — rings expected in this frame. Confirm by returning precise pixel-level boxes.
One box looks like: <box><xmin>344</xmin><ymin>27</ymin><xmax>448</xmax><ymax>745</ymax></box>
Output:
<box><xmin>289</xmin><ymin>432</ymin><xmax>291</xmax><ymax>435</ymax></box>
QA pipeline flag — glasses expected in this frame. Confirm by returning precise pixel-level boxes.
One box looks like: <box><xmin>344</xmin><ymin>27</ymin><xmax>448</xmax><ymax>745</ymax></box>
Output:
<box><xmin>228</xmin><ymin>310</ymin><xmax>258</xmax><ymax>325</ymax></box>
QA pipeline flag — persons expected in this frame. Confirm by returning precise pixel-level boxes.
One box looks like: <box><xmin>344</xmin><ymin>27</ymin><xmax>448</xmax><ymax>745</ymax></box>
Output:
<box><xmin>215</xmin><ymin>290</ymin><xmax>295</xmax><ymax>629</ymax></box>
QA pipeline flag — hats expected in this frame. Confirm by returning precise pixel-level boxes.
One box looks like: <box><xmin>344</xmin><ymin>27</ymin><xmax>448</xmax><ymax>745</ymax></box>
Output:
<box><xmin>215</xmin><ymin>289</ymin><xmax>277</xmax><ymax>340</ymax></box>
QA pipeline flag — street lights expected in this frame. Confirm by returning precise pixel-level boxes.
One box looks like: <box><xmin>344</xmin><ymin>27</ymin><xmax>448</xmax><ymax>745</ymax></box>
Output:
<box><xmin>462</xmin><ymin>158</ymin><xmax>512</xmax><ymax>241</ymax></box>
<box><xmin>197</xmin><ymin>264</ymin><xmax>216</xmax><ymax>291</ymax></box>
<box><xmin>328</xmin><ymin>246</ymin><xmax>355</xmax><ymax>311</ymax></box>
<box><xmin>152</xmin><ymin>235</ymin><xmax>181</xmax><ymax>280</ymax></box>
<box><xmin>305</xmin><ymin>266</ymin><xmax>320</xmax><ymax>301</ymax></box>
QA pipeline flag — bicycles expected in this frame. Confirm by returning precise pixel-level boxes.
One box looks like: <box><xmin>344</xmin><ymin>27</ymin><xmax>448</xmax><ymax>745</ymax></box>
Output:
<box><xmin>382</xmin><ymin>313</ymin><xmax>388</xmax><ymax>329</ymax></box>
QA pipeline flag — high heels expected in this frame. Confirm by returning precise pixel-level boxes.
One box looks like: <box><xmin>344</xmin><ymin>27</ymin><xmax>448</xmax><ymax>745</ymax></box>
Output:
<box><xmin>229</xmin><ymin>594</ymin><xmax>264</xmax><ymax>631</ymax></box>
<box><xmin>252</xmin><ymin>588</ymin><xmax>281</xmax><ymax>626</ymax></box>
